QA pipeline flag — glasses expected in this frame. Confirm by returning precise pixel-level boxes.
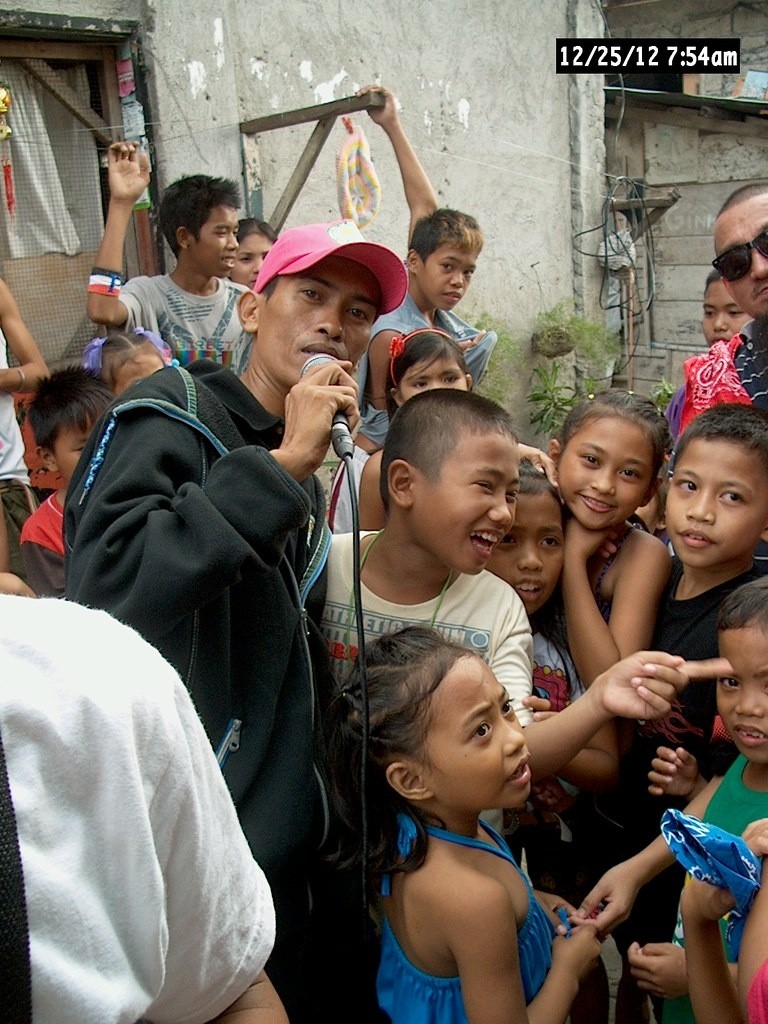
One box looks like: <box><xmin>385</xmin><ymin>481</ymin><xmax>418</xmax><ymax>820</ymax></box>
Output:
<box><xmin>711</xmin><ymin>230</ymin><xmax>768</xmax><ymax>282</ymax></box>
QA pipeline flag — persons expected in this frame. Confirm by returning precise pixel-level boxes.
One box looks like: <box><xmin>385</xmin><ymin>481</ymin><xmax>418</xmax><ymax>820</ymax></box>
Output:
<box><xmin>60</xmin><ymin>220</ymin><xmax>410</xmax><ymax>1024</ymax></box>
<box><xmin>0</xmin><ymin>84</ymin><xmax>768</xmax><ymax>1024</ymax></box>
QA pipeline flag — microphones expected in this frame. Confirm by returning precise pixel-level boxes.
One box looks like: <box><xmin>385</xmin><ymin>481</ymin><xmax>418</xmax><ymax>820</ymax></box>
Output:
<box><xmin>299</xmin><ymin>352</ymin><xmax>354</xmax><ymax>461</ymax></box>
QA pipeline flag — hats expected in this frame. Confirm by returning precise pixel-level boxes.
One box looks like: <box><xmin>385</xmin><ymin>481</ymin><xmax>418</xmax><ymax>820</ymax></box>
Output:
<box><xmin>254</xmin><ymin>220</ymin><xmax>410</xmax><ymax>325</ymax></box>
